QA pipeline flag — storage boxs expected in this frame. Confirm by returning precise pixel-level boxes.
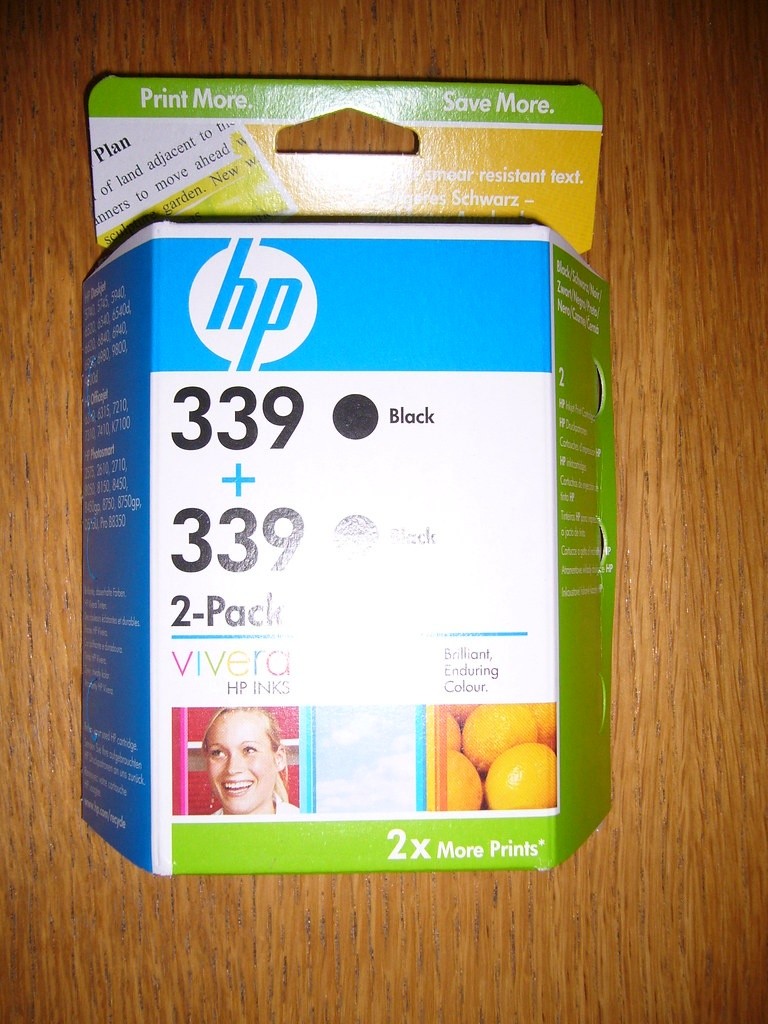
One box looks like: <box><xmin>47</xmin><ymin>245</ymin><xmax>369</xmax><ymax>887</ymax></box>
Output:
<box><xmin>79</xmin><ymin>77</ymin><xmax>619</xmax><ymax>874</ymax></box>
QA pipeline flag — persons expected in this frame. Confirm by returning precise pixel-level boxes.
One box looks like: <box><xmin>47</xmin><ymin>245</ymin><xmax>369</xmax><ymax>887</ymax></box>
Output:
<box><xmin>204</xmin><ymin>707</ymin><xmax>299</xmax><ymax>816</ymax></box>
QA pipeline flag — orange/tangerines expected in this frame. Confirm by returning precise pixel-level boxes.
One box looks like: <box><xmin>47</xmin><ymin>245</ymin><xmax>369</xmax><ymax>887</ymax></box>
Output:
<box><xmin>440</xmin><ymin>703</ymin><xmax>557</xmax><ymax>811</ymax></box>
